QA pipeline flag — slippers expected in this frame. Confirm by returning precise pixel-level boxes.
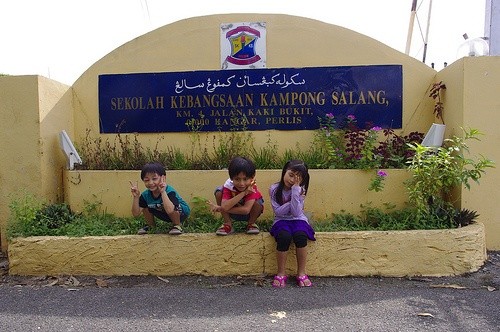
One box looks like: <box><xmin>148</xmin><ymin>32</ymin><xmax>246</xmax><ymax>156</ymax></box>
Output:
<box><xmin>295</xmin><ymin>274</ymin><xmax>312</xmax><ymax>287</ymax></box>
<box><xmin>272</xmin><ymin>274</ymin><xmax>289</xmax><ymax>288</ymax></box>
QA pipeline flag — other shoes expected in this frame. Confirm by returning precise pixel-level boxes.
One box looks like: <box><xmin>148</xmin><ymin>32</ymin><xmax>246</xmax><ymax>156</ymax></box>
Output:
<box><xmin>245</xmin><ymin>223</ymin><xmax>260</xmax><ymax>234</ymax></box>
<box><xmin>168</xmin><ymin>225</ymin><xmax>184</xmax><ymax>235</ymax></box>
<box><xmin>215</xmin><ymin>223</ymin><xmax>235</xmax><ymax>236</ymax></box>
<box><xmin>137</xmin><ymin>225</ymin><xmax>161</xmax><ymax>235</ymax></box>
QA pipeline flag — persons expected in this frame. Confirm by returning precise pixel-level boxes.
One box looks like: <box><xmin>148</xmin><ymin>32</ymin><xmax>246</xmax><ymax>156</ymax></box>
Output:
<box><xmin>129</xmin><ymin>162</ymin><xmax>190</xmax><ymax>235</ymax></box>
<box><xmin>206</xmin><ymin>157</ymin><xmax>264</xmax><ymax>235</ymax></box>
<box><xmin>268</xmin><ymin>160</ymin><xmax>316</xmax><ymax>287</ymax></box>
